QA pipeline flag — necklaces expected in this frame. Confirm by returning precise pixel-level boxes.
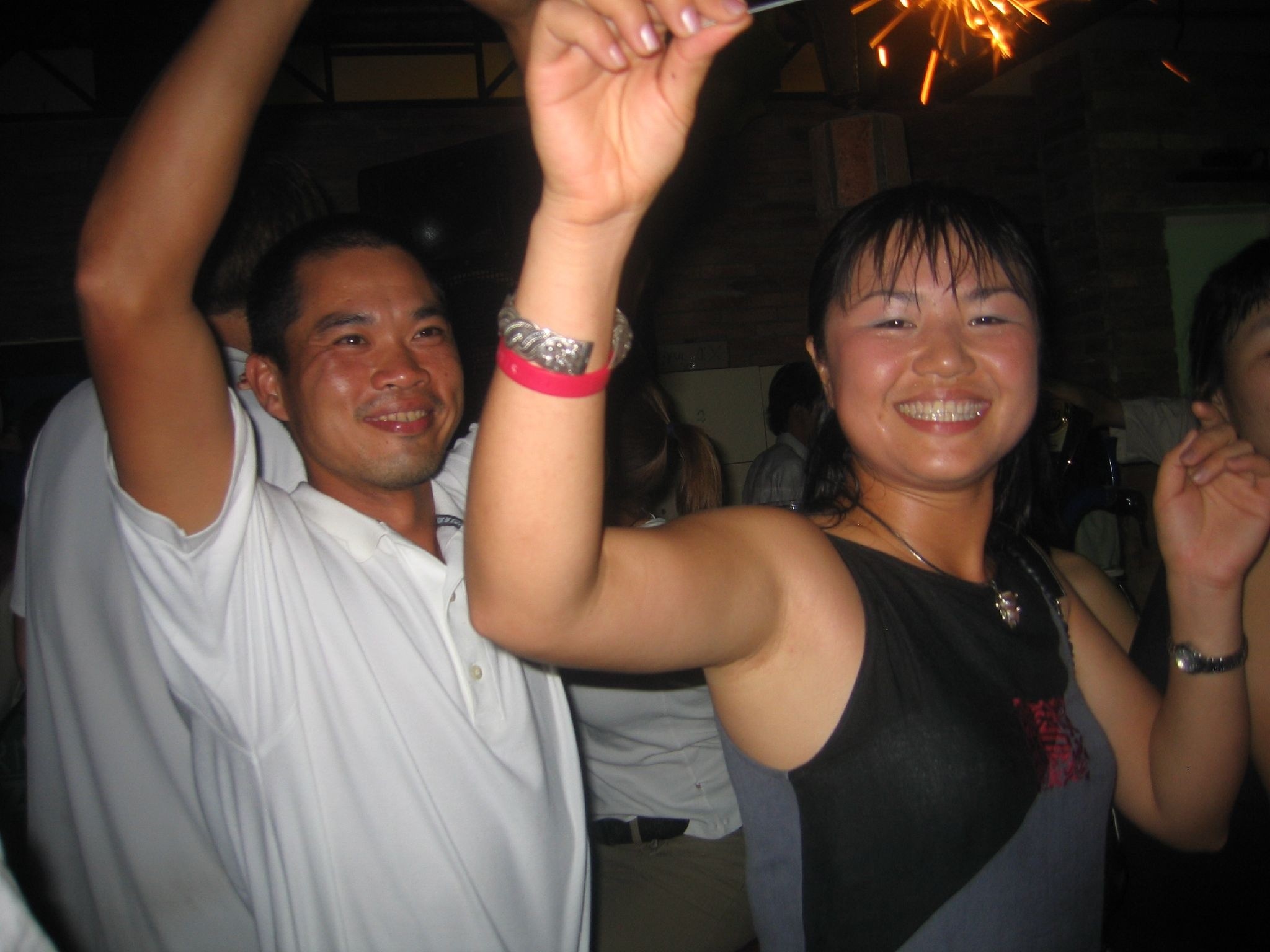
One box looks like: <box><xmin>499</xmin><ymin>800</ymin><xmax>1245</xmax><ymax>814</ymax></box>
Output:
<box><xmin>841</xmin><ymin>487</ymin><xmax>1021</xmax><ymax>631</ymax></box>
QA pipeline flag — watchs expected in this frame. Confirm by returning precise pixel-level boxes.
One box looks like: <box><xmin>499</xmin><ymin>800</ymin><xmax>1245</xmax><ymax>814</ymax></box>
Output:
<box><xmin>1165</xmin><ymin>634</ymin><xmax>1251</xmax><ymax>675</ymax></box>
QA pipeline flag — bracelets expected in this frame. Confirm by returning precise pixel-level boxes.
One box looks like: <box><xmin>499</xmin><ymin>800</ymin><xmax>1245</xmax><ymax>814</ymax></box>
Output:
<box><xmin>496</xmin><ymin>290</ymin><xmax>634</xmax><ymax>374</ymax></box>
<box><xmin>491</xmin><ymin>339</ymin><xmax>617</xmax><ymax>398</ymax></box>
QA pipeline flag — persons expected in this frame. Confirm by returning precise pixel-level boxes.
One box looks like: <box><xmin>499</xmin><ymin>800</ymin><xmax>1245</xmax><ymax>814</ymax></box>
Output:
<box><xmin>0</xmin><ymin>0</ymin><xmax>1270</xmax><ymax>952</ymax></box>
